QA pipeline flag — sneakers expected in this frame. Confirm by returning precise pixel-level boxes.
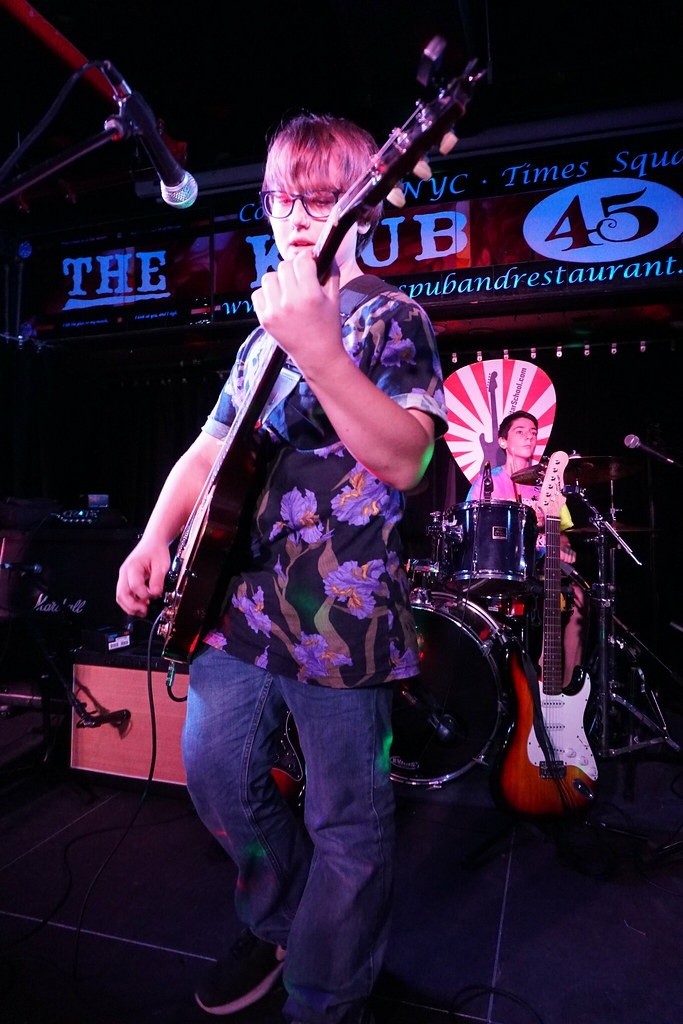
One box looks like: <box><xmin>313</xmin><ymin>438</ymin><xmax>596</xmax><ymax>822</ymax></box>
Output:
<box><xmin>193</xmin><ymin>921</ymin><xmax>290</xmax><ymax>1014</ymax></box>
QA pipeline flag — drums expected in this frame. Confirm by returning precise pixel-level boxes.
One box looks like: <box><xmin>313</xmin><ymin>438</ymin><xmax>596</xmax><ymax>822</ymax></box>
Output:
<box><xmin>406</xmin><ymin>556</ymin><xmax>442</xmax><ymax>592</ymax></box>
<box><xmin>386</xmin><ymin>586</ymin><xmax>512</xmax><ymax>789</ymax></box>
<box><xmin>436</xmin><ymin>498</ymin><xmax>538</xmax><ymax>594</ymax></box>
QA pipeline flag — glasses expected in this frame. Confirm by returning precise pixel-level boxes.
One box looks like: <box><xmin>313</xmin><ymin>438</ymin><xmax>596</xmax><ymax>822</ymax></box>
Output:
<box><xmin>257</xmin><ymin>189</ymin><xmax>353</xmax><ymax>220</ymax></box>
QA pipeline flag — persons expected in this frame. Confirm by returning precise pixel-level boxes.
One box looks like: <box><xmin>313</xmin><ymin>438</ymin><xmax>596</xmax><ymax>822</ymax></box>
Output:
<box><xmin>465</xmin><ymin>411</ymin><xmax>582</xmax><ymax>688</ymax></box>
<box><xmin>116</xmin><ymin>114</ymin><xmax>449</xmax><ymax>1024</ymax></box>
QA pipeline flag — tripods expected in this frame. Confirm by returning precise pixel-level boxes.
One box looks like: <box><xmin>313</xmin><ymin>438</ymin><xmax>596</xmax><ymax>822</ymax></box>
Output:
<box><xmin>562</xmin><ymin>480</ymin><xmax>683</xmax><ymax>796</ymax></box>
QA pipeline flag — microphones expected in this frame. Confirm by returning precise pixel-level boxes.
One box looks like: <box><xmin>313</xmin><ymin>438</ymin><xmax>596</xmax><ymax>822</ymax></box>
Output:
<box><xmin>76</xmin><ymin>710</ymin><xmax>130</xmax><ymax>729</ymax></box>
<box><xmin>1</xmin><ymin>563</ymin><xmax>43</xmax><ymax>574</ymax></box>
<box><xmin>100</xmin><ymin>61</ymin><xmax>198</xmax><ymax>209</ymax></box>
<box><xmin>623</xmin><ymin>434</ymin><xmax>674</xmax><ymax>464</ymax></box>
<box><xmin>434</xmin><ymin>714</ymin><xmax>456</xmax><ymax>739</ymax></box>
<box><xmin>485</xmin><ymin>462</ymin><xmax>494</xmax><ymax>494</ymax></box>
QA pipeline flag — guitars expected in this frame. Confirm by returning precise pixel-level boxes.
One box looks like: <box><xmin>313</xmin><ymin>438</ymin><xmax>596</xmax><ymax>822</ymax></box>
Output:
<box><xmin>495</xmin><ymin>450</ymin><xmax>602</xmax><ymax>819</ymax></box>
<box><xmin>152</xmin><ymin>32</ymin><xmax>492</xmax><ymax>664</ymax></box>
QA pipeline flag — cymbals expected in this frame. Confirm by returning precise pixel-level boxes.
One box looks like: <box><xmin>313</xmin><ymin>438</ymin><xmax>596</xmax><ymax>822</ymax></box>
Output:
<box><xmin>560</xmin><ymin>521</ymin><xmax>661</xmax><ymax>534</ymax></box>
<box><xmin>509</xmin><ymin>454</ymin><xmax>634</xmax><ymax>486</ymax></box>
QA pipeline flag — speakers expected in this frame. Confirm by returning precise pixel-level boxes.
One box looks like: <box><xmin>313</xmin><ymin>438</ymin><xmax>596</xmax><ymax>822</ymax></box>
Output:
<box><xmin>22</xmin><ymin>527</ymin><xmax>146</xmax><ymax>628</ymax></box>
<box><xmin>66</xmin><ymin>646</ymin><xmax>191</xmax><ymax>787</ymax></box>
